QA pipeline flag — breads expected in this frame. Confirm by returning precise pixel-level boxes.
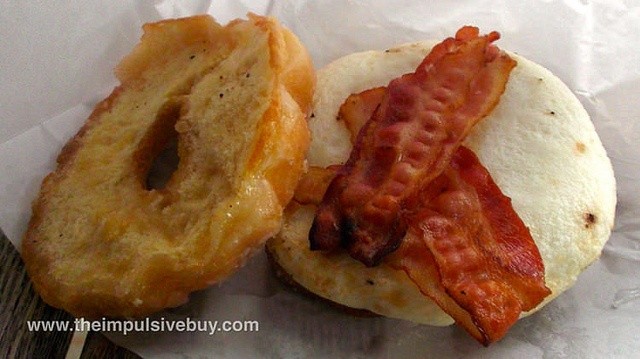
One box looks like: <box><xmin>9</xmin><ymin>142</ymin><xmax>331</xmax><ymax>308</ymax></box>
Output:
<box><xmin>260</xmin><ymin>25</ymin><xmax>618</xmax><ymax>329</ymax></box>
<box><xmin>22</xmin><ymin>13</ymin><xmax>317</xmax><ymax>348</ymax></box>
